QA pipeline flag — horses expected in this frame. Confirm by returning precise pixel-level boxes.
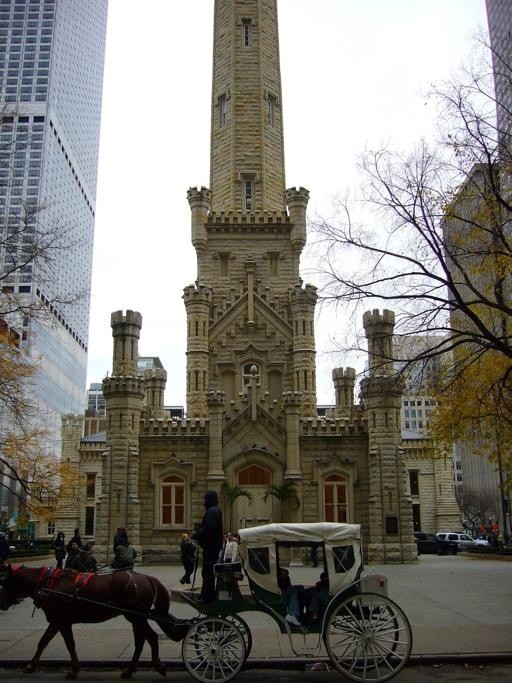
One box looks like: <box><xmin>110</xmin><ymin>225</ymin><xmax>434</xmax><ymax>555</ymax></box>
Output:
<box><xmin>1</xmin><ymin>560</ymin><xmax>231</xmax><ymax>680</ymax></box>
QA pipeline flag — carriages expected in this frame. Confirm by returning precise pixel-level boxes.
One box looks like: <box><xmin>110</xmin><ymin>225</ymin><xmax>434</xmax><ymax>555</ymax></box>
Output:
<box><xmin>0</xmin><ymin>523</ymin><xmax>414</xmax><ymax>682</ymax></box>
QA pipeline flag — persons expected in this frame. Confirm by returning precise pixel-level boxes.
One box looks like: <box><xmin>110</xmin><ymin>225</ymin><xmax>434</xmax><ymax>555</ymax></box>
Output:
<box><xmin>188</xmin><ymin>488</ymin><xmax>225</xmax><ymax>604</ymax></box>
<box><xmin>63</xmin><ymin>542</ymin><xmax>82</xmax><ymax>568</ymax></box>
<box><xmin>178</xmin><ymin>532</ymin><xmax>196</xmax><ymax>585</ymax></box>
<box><xmin>257</xmin><ymin>549</ymin><xmax>301</xmax><ymax>626</ymax></box>
<box><xmin>109</xmin><ymin>535</ymin><xmax>139</xmax><ymax>571</ymax></box>
<box><xmin>217</xmin><ymin>530</ymin><xmax>241</xmax><ymax>562</ymax></box>
<box><xmin>112</xmin><ymin>528</ymin><xmax>125</xmax><ymax>552</ymax></box>
<box><xmin>306</xmin><ymin>547</ymin><xmax>349</xmax><ymax>622</ymax></box>
<box><xmin>52</xmin><ymin>530</ymin><xmax>66</xmax><ymax>572</ymax></box>
<box><xmin>67</xmin><ymin>528</ymin><xmax>82</xmax><ymax>555</ymax></box>
<box><xmin>70</xmin><ymin>545</ymin><xmax>99</xmax><ymax>571</ymax></box>
<box><xmin>123</xmin><ymin>526</ymin><xmax>132</xmax><ymax>549</ymax></box>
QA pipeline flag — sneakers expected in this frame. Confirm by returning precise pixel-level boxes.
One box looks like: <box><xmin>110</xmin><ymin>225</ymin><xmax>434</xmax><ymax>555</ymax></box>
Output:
<box><xmin>180</xmin><ymin>578</ymin><xmax>190</xmax><ymax>584</ymax></box>
<box><xmin>197</xmin><ymin>598</ymin><xmax>213</xmax><ymax>604</ymax></box>
<box><xmin>286</xmin><ymin>615</ymin><xmax>301</xmax><ymax>626</ymax></box>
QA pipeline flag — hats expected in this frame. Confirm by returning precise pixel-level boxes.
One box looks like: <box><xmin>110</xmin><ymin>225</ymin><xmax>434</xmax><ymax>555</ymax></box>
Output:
<box><xmin>204</xmin><ymin>491</ymin><xmax>218</xmax><ymax>499</ymax></box>
<box><xmin>181</xmin><ymin>534</ymin><xmax>188</xmax><ymax>541</ymax></box>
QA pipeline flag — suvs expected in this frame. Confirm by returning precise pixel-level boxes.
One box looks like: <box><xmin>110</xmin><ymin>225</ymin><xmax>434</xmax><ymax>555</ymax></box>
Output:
<box><xmin>436</xmin><ymin>532</ymin><xmax>490</xmax><ymax>553</ymax></box>
<box><xmin>413</xmin><ymin>531</ymin><xmax>457</xmax><ymax>555</ymax></box>
